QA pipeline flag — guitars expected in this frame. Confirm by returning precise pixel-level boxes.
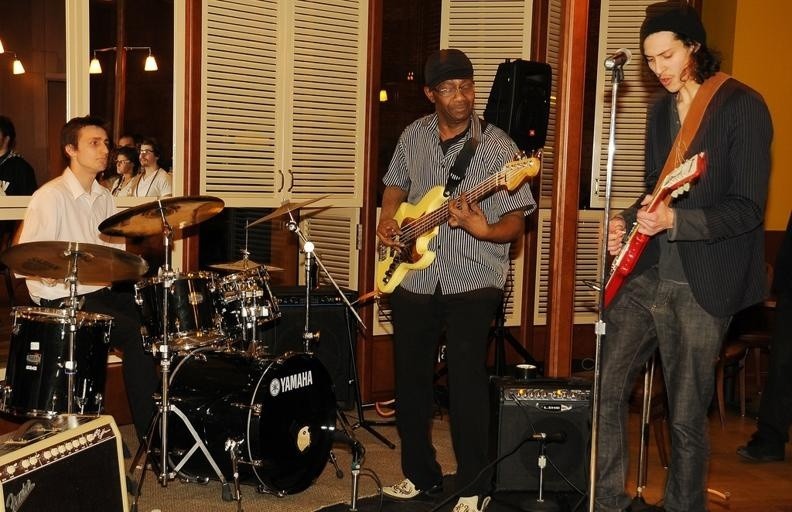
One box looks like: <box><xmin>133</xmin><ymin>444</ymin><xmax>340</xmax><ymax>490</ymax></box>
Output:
<box><xmin>603</xmin><ymin>150</ymin><xmax>707</xmax><ymax>310</ymax></box>
<box><xmin>373</xmin><ymin>149</ymin><xmax>542</xmax><ymax>294</ymax></box>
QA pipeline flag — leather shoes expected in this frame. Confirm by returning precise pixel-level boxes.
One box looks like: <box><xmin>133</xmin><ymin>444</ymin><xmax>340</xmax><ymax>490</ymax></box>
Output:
<box><xmin>378</xmin><ymin>477</ymin><xmax>443</xmax><ymax>499</ymax></box>
<box><xmin>451</xmin><ymin>495</ymin><xmax>491</xmax><ymax>512</ymax></box>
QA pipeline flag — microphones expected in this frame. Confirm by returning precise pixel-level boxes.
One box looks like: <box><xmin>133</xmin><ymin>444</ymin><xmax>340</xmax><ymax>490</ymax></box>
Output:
<box><xmin>605</xmin><ymin>46</ymin><xmax>632</xmax><ymax>73</ymax></box>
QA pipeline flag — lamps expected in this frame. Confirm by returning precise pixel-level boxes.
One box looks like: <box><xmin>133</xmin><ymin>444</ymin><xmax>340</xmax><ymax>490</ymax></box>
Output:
<box><xmin>0</xmin><ymin>38</ymin><xmax>25</xmax><ymax>75</ymax></box>
<box><xmin>89</xmin><ymin>47</ymin><xmax>158</xmax><ymax>75</ymax></box>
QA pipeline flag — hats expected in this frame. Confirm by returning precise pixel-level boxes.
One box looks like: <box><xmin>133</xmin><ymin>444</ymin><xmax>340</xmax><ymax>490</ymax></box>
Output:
<box><xmin>640</xmin><ymin>1</ymin><xmax>706</xmax><ymax>62</ymax></box>
<box><xmin>424</xmin><ymin>49</ymin><xmax>473</xmax><ymax>92</ymax></box>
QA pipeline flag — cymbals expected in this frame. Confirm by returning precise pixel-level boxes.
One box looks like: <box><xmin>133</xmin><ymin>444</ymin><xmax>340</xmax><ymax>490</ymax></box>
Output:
<box><xmin>207</xmin><ymin>257</ymin><xmax>287</xmax><ymax>273</ymax></box>
<box><xmin>98</xmin><ymin>194</ymin><xmax>225</xmax><ymax>238</ymax></box>
<box><xmin>1</xmin><ymin>240</ymin><xmax>149</xmax><ymax>285</ymax></box>
<box><xmin>244</xmin><ymin>195</ymin><xmax>332</xmax><ymax>230</ymax></box>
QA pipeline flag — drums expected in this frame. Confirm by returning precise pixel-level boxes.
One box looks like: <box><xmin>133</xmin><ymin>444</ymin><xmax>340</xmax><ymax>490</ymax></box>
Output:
<box><xmin>133</xmin><ymin>270</ymin><xmax>220</xmax><ymax>343</ymax></box>
<box><xmin>5</xmin><ymin>306</ymin><xmax>114</xmax><ymax>418</ymax></box>
<box><xmin>159</xmin><ymin>344</ymin><xmax>337</xmax><ymax>496</ymax></box>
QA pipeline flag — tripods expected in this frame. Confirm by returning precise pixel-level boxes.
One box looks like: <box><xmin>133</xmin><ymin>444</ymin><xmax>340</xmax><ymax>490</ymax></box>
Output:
<box><xmin>127</xmin><ymin>240</ymin><xmax>235</xmax><ymax>512</ymax></box>
<box><xmin>343</xmin><ymin>305</ymin><xmax>400</xmax><ymax>451</ymax></box>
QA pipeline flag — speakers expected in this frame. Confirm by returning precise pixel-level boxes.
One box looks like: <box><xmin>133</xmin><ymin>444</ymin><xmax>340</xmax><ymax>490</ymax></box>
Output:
<box><xmin>0</xmin><ymin>410</ymin><xmax>126</xmax><ymax>512</ymax></box>
<box><xmin>487</xmin><ymin>372</ymin><xmax>593</xmax><ymax>511</ymax></box>
<box><xmin>482</xmin><ymin>55</ymin><xmax>552</xmax><ymax>152</ymax></box>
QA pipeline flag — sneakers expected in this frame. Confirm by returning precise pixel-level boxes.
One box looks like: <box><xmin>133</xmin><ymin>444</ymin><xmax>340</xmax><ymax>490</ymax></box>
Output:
<box><xmin>736</xmin><ymin>445</ymin><xmax>785</xmax><ymax>463</ymax></box>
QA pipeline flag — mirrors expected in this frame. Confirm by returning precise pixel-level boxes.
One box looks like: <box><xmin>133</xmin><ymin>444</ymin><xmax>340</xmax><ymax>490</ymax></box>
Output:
<box><xmin>0</xmin><ymin>0</ymin><xmax>65</xmax><ymax>196</ymax></box>
<box><xmin>89</xmin><ymin>0</ymin><xmax>174</xmax><ymax>197</ymax></box>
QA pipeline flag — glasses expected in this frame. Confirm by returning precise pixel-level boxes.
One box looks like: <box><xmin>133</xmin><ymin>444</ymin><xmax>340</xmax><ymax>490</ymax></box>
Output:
<box><xmin>137</xmin><ymin>149</ymin><xmax>154</xmax><ymax>155</ymax></box>
<box><xmin>434</xmin><ymin>81</ymin><xmax>474</xmax><ymax>96</ymax></box>
<box><xmin>114</xmin><ymin>160</ymin><xmax>131</xmax><ymax>166</ymax></box>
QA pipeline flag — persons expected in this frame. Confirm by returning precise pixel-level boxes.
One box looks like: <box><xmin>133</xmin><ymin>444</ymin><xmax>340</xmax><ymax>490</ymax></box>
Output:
<box><xmin>95</xmin><ymin>129</ymin><xmax>173</xmax><ymax>199</ymax></box>
<box><xmin>0</xmin><ymin>115</ymin><xmax>38</xmax><ymax>197</ymax></box>
<box><xmin>733</xmin><ymin>216</ymin><xmax>791</xmax><ymax>464</ymax></box>
<box><xmin>583</xmin><ymin>3</ymin><xmax>775</xmax><ymax>511</ymax></box>
<box><xmin>15</xmin><ymin>115</ymin><xmax>176</xmax><ymax>490</ymax></box>
<box><xmin>372</xmin><ymin>48</ymin><xmax>542</xmax><ymax>511</ymax></box>
